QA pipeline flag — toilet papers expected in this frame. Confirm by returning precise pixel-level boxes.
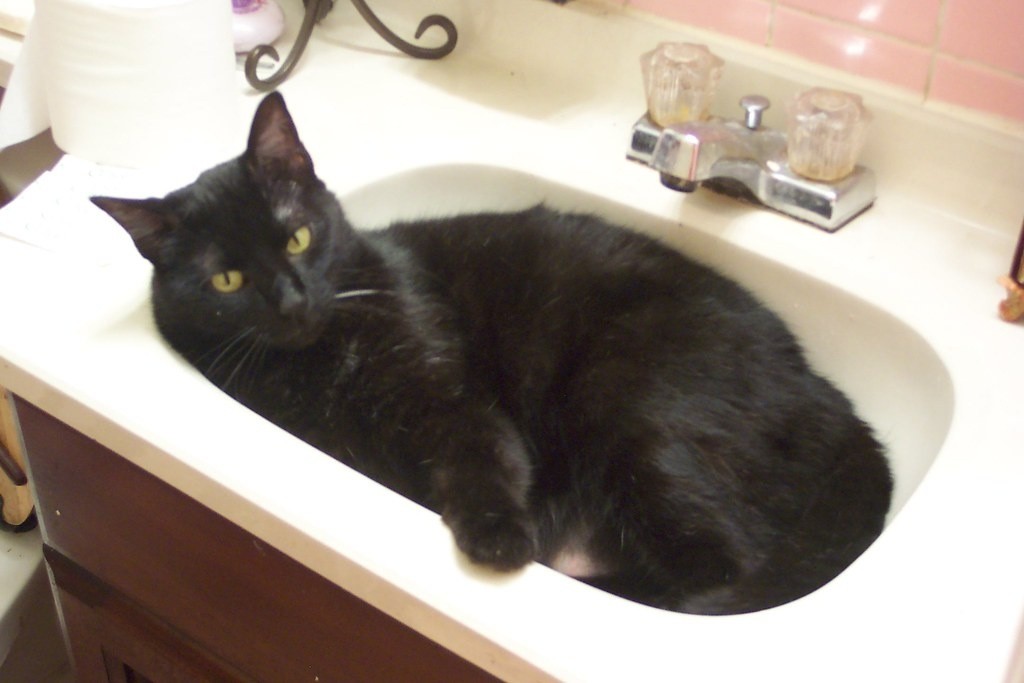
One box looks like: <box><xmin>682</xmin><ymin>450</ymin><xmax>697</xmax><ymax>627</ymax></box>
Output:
<box><xmin>0</xmin><ymin>0</ymin><xmax>237</xmax><ymax>161</ymax></box>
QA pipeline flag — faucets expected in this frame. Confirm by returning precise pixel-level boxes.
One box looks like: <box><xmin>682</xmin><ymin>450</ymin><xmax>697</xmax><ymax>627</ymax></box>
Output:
<box><xmin>648</xmin><ymin>122</ymin><xmax>764</xmax><ymax>193</ymax></box>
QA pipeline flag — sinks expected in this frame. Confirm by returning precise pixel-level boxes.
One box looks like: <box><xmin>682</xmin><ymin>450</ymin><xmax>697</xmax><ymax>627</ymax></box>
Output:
<box><xmin>156</xmin><ymin>163</ymin><xmax>954</xmax><ymax>615</ymax></box>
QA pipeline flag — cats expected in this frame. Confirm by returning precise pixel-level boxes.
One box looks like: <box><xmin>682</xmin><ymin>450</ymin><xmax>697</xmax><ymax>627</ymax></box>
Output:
<box><xmin>86</xmin><ymin>89</ymin><xmax>895</xmax><ymax>618</ymax></box>
